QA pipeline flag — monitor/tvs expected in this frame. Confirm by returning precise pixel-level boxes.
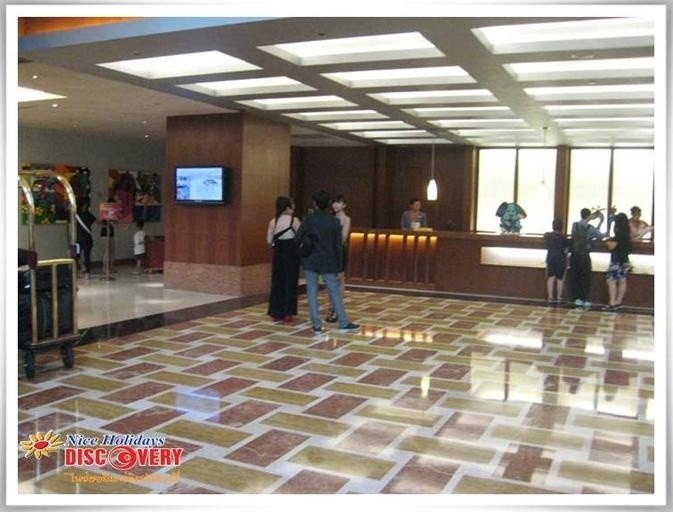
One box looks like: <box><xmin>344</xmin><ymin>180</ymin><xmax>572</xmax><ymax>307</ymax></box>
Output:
<box><xmin>172</xmin><ymin>164</ymin><xmax>225</xmax><ymax>205</ymax></box>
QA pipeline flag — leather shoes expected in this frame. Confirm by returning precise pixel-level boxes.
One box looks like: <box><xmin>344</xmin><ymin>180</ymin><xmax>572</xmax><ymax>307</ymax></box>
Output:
<box><xmin>326</xmin><ymin>309</ymin><xmax>339</xmax><ymax>323</ymax></box>
<box><xmin>273</xmin><ymin>313</ymin><xmax>293</xmax><ymax>325</ymax></box>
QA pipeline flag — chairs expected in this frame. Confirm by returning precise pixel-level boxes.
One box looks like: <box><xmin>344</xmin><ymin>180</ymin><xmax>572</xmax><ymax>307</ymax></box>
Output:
<box><xmin>143</xmin><ymin>235</ymin><xmax>165</xmax><ymax>275</ymax></box>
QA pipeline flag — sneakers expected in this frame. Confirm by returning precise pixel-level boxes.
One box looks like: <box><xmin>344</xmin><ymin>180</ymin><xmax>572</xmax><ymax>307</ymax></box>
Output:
<box><xmin>338</xmin><ymin>322</ymin><xmax>362</xmax><ymax>334</ymax></box>
<box><xmin>574</xmin><ymin>298</ymin><xmax>592</xmax><ymax>308</ymax></box>
<box><xmin>310</xmin><ymin>326</ymin><xmax>323</xmax><ymax>335</ymax></box>
<box><xmin>547</xmin><ymin>297</ymin><xmax>568</xmax><ymax>304</ymax></box>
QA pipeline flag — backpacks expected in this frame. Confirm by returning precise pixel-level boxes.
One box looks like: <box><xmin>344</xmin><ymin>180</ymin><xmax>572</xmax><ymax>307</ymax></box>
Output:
<box><xmin>570</xmin><ymin>223</ymin><xmax>591</xmax><ymax>256</ymax></box>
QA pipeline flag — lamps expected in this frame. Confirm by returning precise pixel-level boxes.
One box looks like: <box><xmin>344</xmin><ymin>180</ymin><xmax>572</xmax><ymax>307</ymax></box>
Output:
<box><xmin>426</xmin><ymin>144</ymin><xmax>438</xmax><ymax>201</ymax></box>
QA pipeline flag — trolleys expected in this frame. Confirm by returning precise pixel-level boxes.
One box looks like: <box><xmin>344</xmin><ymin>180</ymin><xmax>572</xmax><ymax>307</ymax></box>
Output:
<box><xmin>18</xmin><ymin>169</ymin><xmax>84</xmax><ymax>379</ymax></box>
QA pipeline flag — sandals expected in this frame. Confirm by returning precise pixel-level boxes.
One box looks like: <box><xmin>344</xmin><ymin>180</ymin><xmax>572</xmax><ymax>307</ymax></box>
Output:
<box><xmin>600</xmin><ymin>302</ymin><xmax>624</xmax><ymax>312</ymax></box>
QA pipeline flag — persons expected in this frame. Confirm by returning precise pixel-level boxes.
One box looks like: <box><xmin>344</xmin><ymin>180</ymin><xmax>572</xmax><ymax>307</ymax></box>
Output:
<box><xmin>565</xmin><ymin>208</ymin><xmax>608</xmax><ymax>308</ymax></box>
<box><xmin>627</xmin><ymin>204</ymin><xmax>653</xmax><ymax>240</ymax></box>
<box><xmin>72</xmin><ymin>195</ymin><xmax>109</xmax><ymax>282</ymax></box>
<box><xmin>600</xmin><ymin>212</ymin><xmax>632</xmax><ymax>312</ymax></box>
<box><xmin>295</xmin><ymin>190</ymin><xmax>361</xmax><ymax>334</ymax></box>
<box><xmin>132</xmin><ymin>218</ymin><xmax>148</xmax><ymax>274</ymax></box>
<box><xmin>324</xmin><ymin>192</ymin><xmax>351</xmax><ymax>323</ymax></box>
<box><xmin>265</xmin><ymin>196</ymin><xmax>304</xmax><ymax>323</ymax></box>
<box><xmin>399</xmin><ymin>197</ymin><xmax>427</xmax><ymax>230</ymax></box>
<box><xmin>542</xmin><ymin>217</ymin><xmax>569</xmax><ymax>305</ymax></box>
